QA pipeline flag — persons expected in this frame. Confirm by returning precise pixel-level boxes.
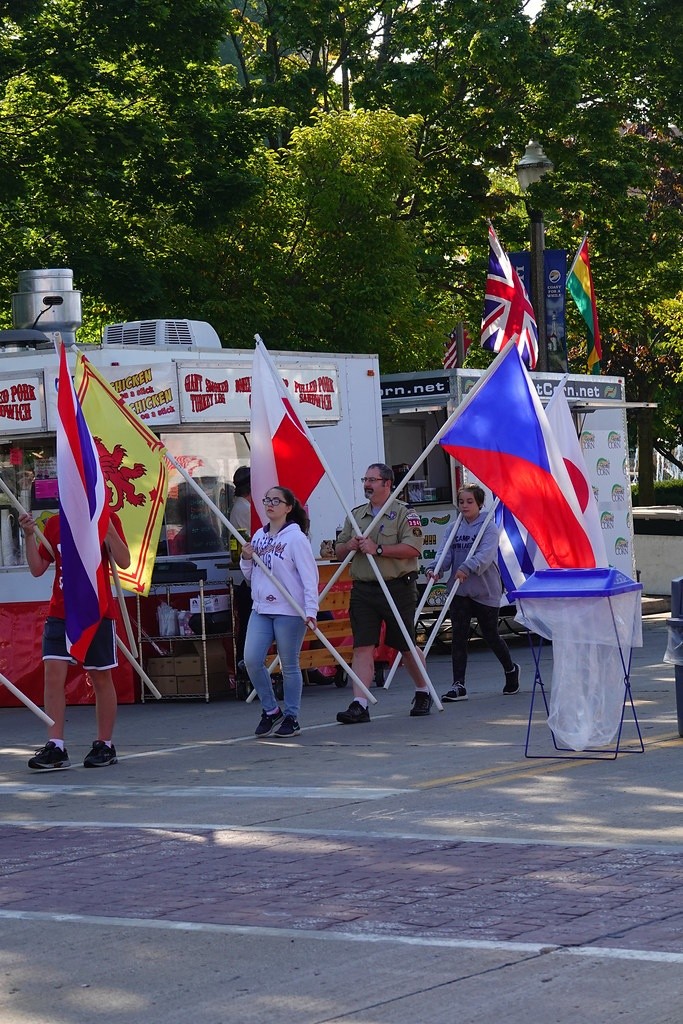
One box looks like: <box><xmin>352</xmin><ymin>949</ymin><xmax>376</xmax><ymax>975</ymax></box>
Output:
<box><xmin>239</xmin><ymin>487</ymin><xmax>319</xmax><ymax>737</ymax></box>
<box><xmin>333</xmin><ymin>463</ymin><xmax>434</xmax><ymax>724</ymax></box>
<box><xmin>229</xmin><ymin>466</ymin><xmax>251</xmax><ymax>547</ymax></box>
<box><xmin>18</xmin><ymin>510</ymin><xmax>130</xmax><ymax>769</ymax></box>
<box><xmin>425</xmin><ymin>482</ymin><xmax>521</xmax><ymax>700</ymax></box>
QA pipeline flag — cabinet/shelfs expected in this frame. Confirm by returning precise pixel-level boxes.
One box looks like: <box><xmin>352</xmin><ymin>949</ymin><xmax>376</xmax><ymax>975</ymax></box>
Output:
<box><xmin>134</xmin><ymin>578</ymin><xmax>239</xmax><ymax>703</ymax></box>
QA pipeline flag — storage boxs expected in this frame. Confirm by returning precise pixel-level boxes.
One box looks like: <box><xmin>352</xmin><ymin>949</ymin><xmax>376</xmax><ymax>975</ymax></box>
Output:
<box><xmin>407</xmin><ymin>479</ymin><xmax>427</xmax><ymax>502</ymax></box>
<box><xmin>145</xmin><ymin>593</ymin><xmax>232</xmax><ymax>693</ymax></box>
<box><xmin>423</xmin><ymin>487</ymin><xmax>438</xmax><ymax>501</ymax></box>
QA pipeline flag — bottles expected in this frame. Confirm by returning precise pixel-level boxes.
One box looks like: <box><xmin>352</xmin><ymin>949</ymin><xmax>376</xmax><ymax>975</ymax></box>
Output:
<box><xmin>231</xmin><ymin>528</ymin><xmax>251</xmax><ymax>562</ymax></box>
<box><xmin>178</xmin><ymin>610</ymin><xmax>187</xmax><ymax>637</ymax></box>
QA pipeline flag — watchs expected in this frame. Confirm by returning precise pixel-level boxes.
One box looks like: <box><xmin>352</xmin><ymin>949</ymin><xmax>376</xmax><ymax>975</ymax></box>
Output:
<box><xmin>375</xmin><ymin>544</ymin><xmax>383</xmax><ymax>556</ymax></box>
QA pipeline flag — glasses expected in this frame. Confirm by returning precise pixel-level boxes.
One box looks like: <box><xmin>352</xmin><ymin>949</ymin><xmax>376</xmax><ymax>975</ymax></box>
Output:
<box><xmin>261</xmin><ymin>496</ymin><xmax>288</xmax><ymax>506</ymax></box>
<box><xmin>360</xmin><ymin>476</ymin><xmax>383</xmax><ymax>484</ymax></box>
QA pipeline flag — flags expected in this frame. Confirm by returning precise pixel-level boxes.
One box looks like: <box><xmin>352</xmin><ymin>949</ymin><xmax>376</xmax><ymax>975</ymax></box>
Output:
<box><xmin>479</xmin><ymin>223</ymin><xmax>540</xmax><ymax>370</ymax></box>
<box><xmin>437</xmin><ymin>344</ymin><xmax>610</xmax><ymax>569</ymax></box>
<box><xmin>545</xmin><ymin>387</ymin><xmax>608</xmax><ymax>570</ymax></box>
<box><xmin>250</xmin><ymin>341</ymin><xmax>326</xmax><ymax>542</ymax></box>
<box><xmin>566</xmin><ymin>237</ymin><xmax>603</xmax><ymax>375</ymax></box>
<box><xmin>55</xmin><ymin>341</ymin><xmax>110</xmax><ymax>661</ymax></box>
<box><xmin>73</xmin><ymin>351</ymin><xmax>168</xmax><ymax>597</ymax></box>
<box><xmin>494</xmin><ymin>489</ymin><xmax>550</xmax><ymax>604</ymax></box>
<box><xmin>441</xmin><ymin>326</ymin><xmax>471</xmax><ymax>369</ymax></box>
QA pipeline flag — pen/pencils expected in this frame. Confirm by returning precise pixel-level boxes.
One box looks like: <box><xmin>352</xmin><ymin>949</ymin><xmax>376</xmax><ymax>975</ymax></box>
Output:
<box><xmin>380</xmin><ymin>525</ymin><xmax>384</xmax><ymax>531</ymax></box>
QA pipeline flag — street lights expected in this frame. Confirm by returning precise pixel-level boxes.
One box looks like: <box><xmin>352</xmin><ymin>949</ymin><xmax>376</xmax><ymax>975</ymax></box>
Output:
<box><xmin>514</xmin><ymin>139</ymin><xmax>555</xmax><ymax>372</ymax></box>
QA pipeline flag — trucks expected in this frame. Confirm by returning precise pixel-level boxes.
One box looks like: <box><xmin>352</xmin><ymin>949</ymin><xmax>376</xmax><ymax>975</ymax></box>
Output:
<box><xmin>1</xmin><ymin>269</ymin><xmax>386</xmax><ymax>686</ymax></box>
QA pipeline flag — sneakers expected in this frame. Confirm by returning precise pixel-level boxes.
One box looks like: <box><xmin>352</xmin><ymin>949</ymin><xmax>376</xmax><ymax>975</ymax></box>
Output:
<box><xmin>337</xmin><ymin>701</ymin><xmax>371</xmax><ymax>723</ymax></box>
<box><xmin>28</xmin><ymin>742</ymin><xmax>71</xmax><ymax>769</ymax></box>
<box><xmin>410</xmin><ymin>690</ymin><xmax>434</xmax><ymax>716</ymax></box>
<box><xmin>503</xmin><ymin>663</ymin><xmax>521</xmax><ymax>695</ymax></box>
<box><xmin>441</xmin><ymin>681</ymin><xmax>469</xmax><ymax>701</ymax></box>
<box><xmin>255</xmin><ymin>707</ymin><xmax>284</xmax><ymax>737</ymax></box>
<box><xmin>274</xmin><ymin>716</ymin><xmax>301</xmax><ymax>737</ymax></box>
<box><xmin>84</xmin><ymin>740</ymin><xmax>118</xmax><ymax>766</ymax></box>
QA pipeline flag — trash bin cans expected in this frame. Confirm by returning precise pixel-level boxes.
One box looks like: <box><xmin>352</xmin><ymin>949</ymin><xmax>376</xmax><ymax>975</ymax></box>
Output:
<box><xmin>511</xmin><ymin>565</ymin><xmax>645</xmax><ymax>760</ymax></box>
<box><xmin>666</xmin><ymin>576</ymin><xmax>683</xmax><ymax>737</ymax></box>
